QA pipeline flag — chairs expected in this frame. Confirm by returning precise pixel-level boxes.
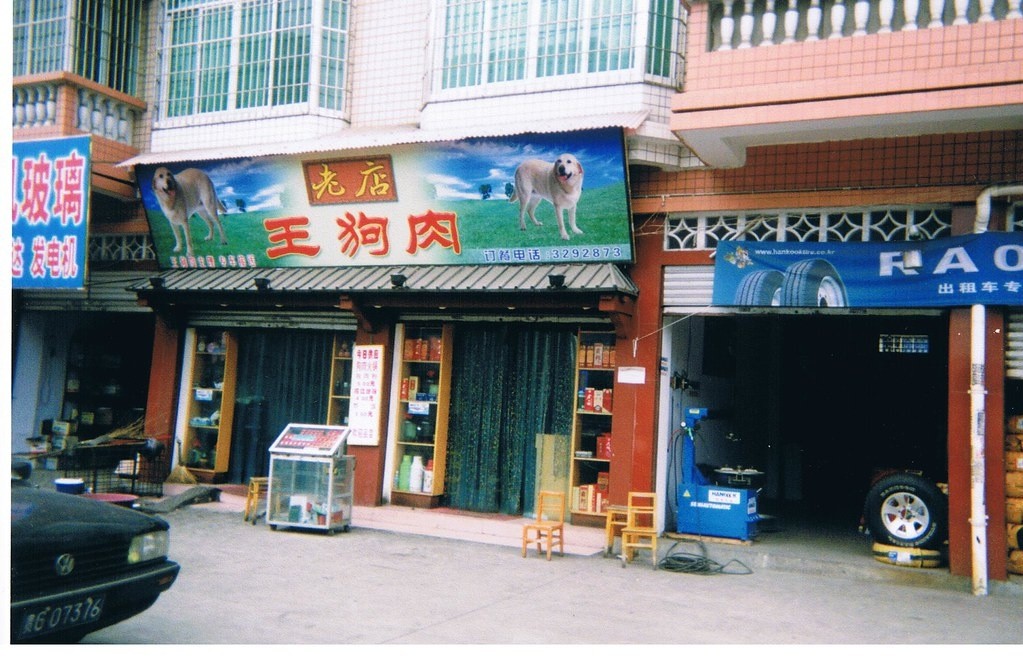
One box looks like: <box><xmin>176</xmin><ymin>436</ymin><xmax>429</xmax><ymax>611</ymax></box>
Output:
<box><xmin>620</xmin><ymin>491</ymin><xmax>657</xmax><ymax>569</ymax></box>
<box><xmin>523</xmin><ymin>490</ymin><xmax>566</xmax><ymax>560</ymax></box>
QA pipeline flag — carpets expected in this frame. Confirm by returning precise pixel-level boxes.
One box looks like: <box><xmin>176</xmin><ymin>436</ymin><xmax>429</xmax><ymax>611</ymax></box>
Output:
<box><xmin>218</xmin><ymin>486</ymin><xmax>279</xmax><ymax>499</ymax></box>
<box><xmin>423</xmin><ymin>506</ymin><xmax>522</xmax><ymax>522</ymax></box>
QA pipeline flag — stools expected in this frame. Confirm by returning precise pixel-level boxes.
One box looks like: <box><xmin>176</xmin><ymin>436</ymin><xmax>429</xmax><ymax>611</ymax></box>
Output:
<box><xmin>604</xmin><ymin>505</ymin><xmax>641</xmax><ymax>559</ymax></box>
<box><xmin>244</xmin><ymin>477</ymin><xmax>281</xmax><ymax>526</ymax></box>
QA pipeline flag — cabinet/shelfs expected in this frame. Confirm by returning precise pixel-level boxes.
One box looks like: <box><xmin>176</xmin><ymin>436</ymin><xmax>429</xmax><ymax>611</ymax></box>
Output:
<box><xmin>326</xmin><ymin>330</ymin><xmax>357</xmax><ymax>431</ymax></box>
<box><xmin>569</xmin><ymin>327</ymin><xmax>616</xmax><ymax>528</ymax></box>
<box><xmin>181</xmin><ymin>327</ymin><xmax>237</xmax><ymax>485</ymax></box>
<box><xmin>391</xmin><ymin>323</ymin><xmax>452</xmax><ymax>508</ymax></box>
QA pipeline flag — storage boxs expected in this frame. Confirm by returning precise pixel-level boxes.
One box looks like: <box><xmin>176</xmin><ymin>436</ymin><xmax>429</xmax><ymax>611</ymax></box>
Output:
<box><xmin>290</xmin><ymin>495</ymin><xmax>342</xmax><ymax>525</ymax></box>
<box><xmin>11</xmin><ymin>408</ymin><xmax>112</xmax><ymax>472</ymax></box>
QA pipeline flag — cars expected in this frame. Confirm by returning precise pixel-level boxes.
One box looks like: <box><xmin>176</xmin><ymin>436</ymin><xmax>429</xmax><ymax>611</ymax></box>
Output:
<box><xmin>11</xmin><ymin>460</ymin><xmax>181</xmax><ymax>645</ymax></box>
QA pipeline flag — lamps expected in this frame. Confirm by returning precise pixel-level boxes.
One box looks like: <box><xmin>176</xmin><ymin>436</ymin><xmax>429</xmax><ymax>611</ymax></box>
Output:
<box><xmin>389</xmin><ymin>273</ymin><xmax>408</xmax><ymax>288</ymax></box>
<box><xmin>547</xmin><ymin>274</ymin><xmax>567</xmax><ymax>287</ymax></box>
<box><xmin>149</xmin><ymin>278</ymin><xmax>165</xmax><ymax>289</ymax></box>
<box><xmin>254</xmin><ymin>277</ymin><xmax>270</xmax><ymax>290</ymax></box>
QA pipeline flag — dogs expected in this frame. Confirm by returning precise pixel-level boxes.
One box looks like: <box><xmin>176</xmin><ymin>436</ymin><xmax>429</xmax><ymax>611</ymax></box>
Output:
<box><xmin>508</xmin><ymin>153</ymin><xmax>584</xmax><ymax>241</ymax></box>
<box><xmin>151</xmin><ymin>166</ymin><xmax>229</xmax><ymax>260</ymax></box>
